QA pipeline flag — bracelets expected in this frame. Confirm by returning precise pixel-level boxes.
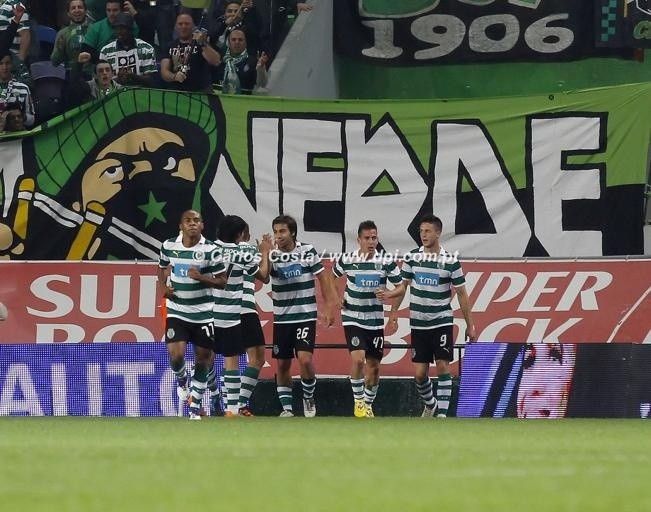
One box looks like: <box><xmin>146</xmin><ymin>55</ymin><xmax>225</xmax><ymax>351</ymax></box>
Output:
<box><xmin>390</xmin><ymin>319</ymin><xmax>399</xmax><ymax>323</ymax></box>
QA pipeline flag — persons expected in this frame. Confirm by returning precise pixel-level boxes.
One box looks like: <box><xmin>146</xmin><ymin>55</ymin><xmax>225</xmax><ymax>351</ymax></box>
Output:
<box><xmin>516</xmin><ymin>343</ymin><xmax>642</xmax><ymax>418</ymax></box>
<box><xmin>157</xmin><ymin>209</ymin><xmax>273</xmax><ymax>421</ymax></box>
<box><xmin>383</xmin><ymin>215</ymin><xmax>479</xmax><ymax>418</ymax></box>
<box><xmin>0</xmin><ymin>0</ymin><xmax>315</xmax><ymax>137</ymax></box>
<box><xmin>261</xmin><ymin>214</ymin><xmax>335</xmax><ymax>417</ymax></box>
<box><xmin>330</xmin><ymin>220</ymin><xmax>408</xmax><ymax>418</ymax></box>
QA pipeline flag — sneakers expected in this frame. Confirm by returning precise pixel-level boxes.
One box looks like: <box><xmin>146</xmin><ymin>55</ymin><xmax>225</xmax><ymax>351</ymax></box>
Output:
<box><xmin>365</xmin><ymin>403</ymin><xmax>375</xmax><ymax>417</ymax></box>
<box><xmin>224</xmin><ymin>410</ymin><xmax>240</xmax><ymax>416</ymax></box>
<box><xmin>302</xmin><ymin>397</ymin><xmax>316</xmax><ymax>417</ymax></box>
<box><xmin>353</xmin><ymin>398</ymin><xmax>365</xmax><ymax>417</ymax></box>
<box><xmin>280</xmin><ymin>409</ymin><xmax>295</xmax><ymax>417</ymax></box>
<box><xmin>421</xmin><ymin>396</ymin><xmax>438</xmax><ymax>417</ymax></box>
<box><xmin>176</xmin><ymin>371</ymin><xmax>190</xmax><ymax>401</ymax></box>
<box><xmin>210</xmin><ymin>395</ymin><xmax>221</xmax><ymax>416</ymax></box>
<box><xmin>239</xmin><ymin>406</ymin><xmax>255</xmax><ymax>416</ymax></box>
<box><xmin>187</xmin><ymin>395</ymin><xmax>207</xmax><ymax>416</ymax></box>
<box><xmin>190</xmin><ymin>413</ymin><xmax>202</xmax><ymax>421</ymax></box>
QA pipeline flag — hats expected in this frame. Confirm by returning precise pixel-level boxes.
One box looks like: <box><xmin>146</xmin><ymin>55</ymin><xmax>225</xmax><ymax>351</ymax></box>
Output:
<box><xmin>21</xmin><ymin>86</ymin><xmax>226</xmax><ymax>214</ymax></box>
<box><xmin>110</xmin><ymin>12</ymin><xmax>133</xmax><ymax>28</ymax></box>
